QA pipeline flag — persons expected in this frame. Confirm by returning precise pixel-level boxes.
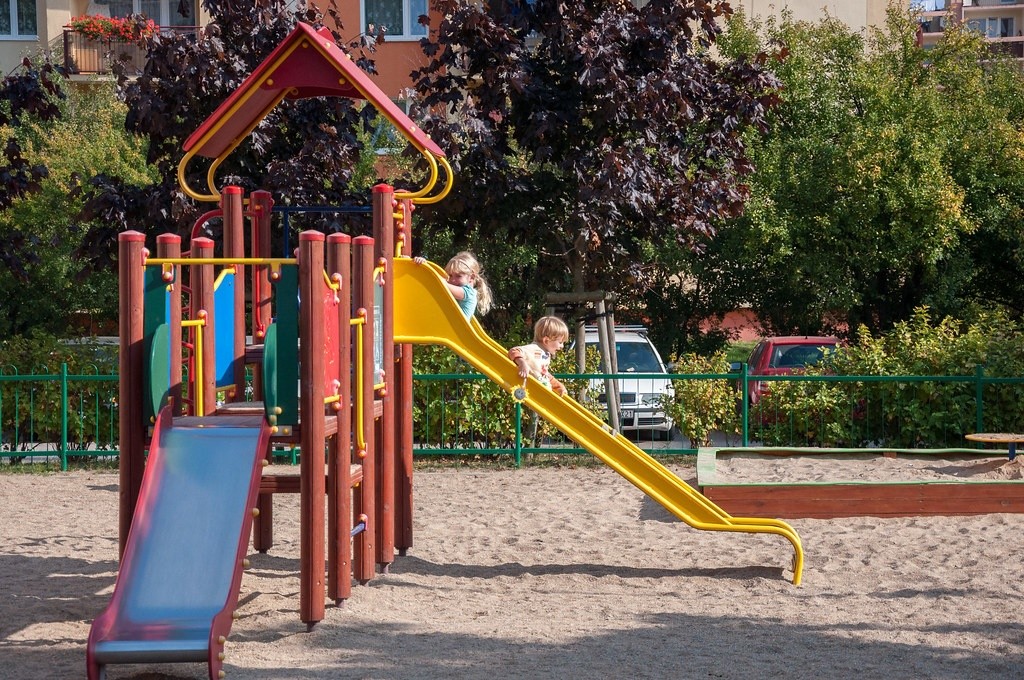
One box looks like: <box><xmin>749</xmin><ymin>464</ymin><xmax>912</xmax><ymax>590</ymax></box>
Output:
<box><xmin>508</xmin><ymin>315</ymin><xmax>616</xmax><ymax>440</ymax></box>
<box><xmin>402</xmin><ymin>249</ymin><xmax>494</xmax><ymax>323</ymax></box>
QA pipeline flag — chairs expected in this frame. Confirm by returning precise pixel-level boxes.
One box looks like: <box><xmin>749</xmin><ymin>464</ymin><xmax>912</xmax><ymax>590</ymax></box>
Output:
<box><xmin>623</xmin><ymin>353</ymin><xmax>651</xmax><ymax>371</ymax></box>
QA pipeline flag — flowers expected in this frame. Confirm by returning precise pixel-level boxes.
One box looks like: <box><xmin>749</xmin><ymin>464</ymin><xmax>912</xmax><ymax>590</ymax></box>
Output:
<box><xmin>67</xmin><ymin>14</ymin><xmax>160</xmax><ymax>50</ymax></box>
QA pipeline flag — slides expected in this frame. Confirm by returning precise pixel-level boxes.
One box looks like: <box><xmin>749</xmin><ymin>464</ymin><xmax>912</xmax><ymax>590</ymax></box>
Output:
<box><xmin>85</xmin><ymin>405</ymin><xmax>272</xmax><ymax>680</ymax></box>
<box><xmin>392</xmin><ymin>254</ymin><xmax>804</xmax><ymax>583</ymax></box>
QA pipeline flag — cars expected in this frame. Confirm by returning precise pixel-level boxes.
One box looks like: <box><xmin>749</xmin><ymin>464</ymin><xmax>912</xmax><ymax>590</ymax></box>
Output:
<box><xmin>729</xmin><ymin>336</ymin><xmax>868</xmax><ymax>430</ymax></box>
<box><xmin>568</xmin><ymin>324</ymin><xmax>675</xmax><ymax>442</ymax></box>
